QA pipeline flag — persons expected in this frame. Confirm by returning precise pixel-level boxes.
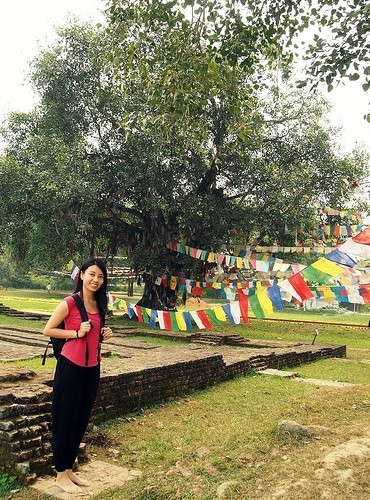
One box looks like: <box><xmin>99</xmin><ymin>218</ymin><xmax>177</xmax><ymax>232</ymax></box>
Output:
<box><xmin>43</xmin><ymin>258</ymin><xmax>113</xmax><ymax>494</ymax></box>
<box><xmin>45</xmin><ymin>282</ymin><xmax>51</xmax><ymax>294</ymax></box>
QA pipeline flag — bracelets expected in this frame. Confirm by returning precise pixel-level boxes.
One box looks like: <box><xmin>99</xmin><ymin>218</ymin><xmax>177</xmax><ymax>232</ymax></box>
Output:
<box><xmin>76</xmin><ymin>330</ymin><xmax>79</xmax><ymax>338</ymax></box>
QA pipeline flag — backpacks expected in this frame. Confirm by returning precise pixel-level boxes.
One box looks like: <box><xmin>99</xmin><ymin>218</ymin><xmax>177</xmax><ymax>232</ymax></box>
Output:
<box><xmin>41</xmin><ymin>294</ymin><xmax>105</xmax><ymax>366</ymax></box>
